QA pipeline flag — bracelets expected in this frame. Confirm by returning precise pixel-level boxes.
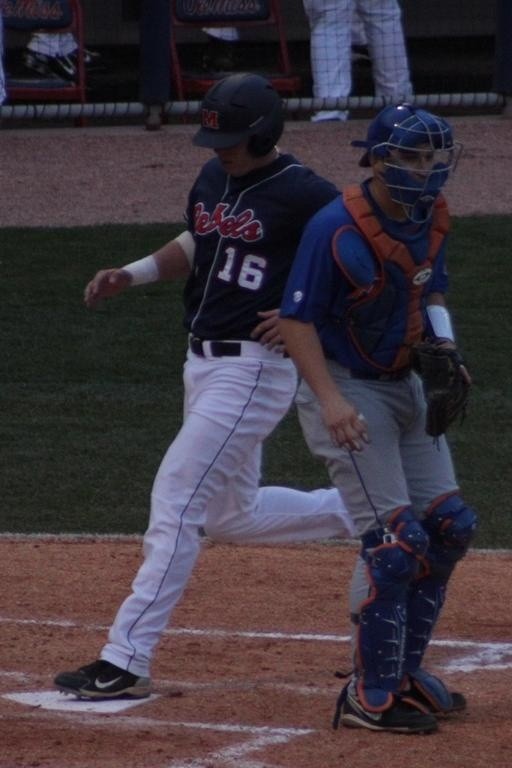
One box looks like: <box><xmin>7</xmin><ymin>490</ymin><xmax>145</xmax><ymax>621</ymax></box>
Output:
<box><xmin>425</xmin><ymin>303</ymin><xmax>456</xmax><ymax>347</ymax></box>
<box><xmin>123</xmin><ymin>254</ymin><xmax>158</xmax><ymax>290</ymax></box>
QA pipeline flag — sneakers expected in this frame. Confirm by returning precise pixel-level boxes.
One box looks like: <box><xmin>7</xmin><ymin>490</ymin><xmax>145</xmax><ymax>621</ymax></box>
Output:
<box><xmin>339</xmin><ymin>690</ymin><xmax>438</xmax><ymax>734</ymax></box>
<box><xmin>401</xmin><ymin>678</ymin><xmax>467</xmax><ymax>720</ymax></box>
<box><xmin>53</xmin><ymin>659</ymin><xmax>154</xmax><ymax>702</ymax></box>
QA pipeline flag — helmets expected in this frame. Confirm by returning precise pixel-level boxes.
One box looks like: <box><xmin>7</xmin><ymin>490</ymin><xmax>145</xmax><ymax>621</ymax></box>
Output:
<box><xmin>188</xmin><ymin>71</ymin><xmax>287</xmax><ymax>158</ymax></box>
<box><xmin>358</xmin><ymin>103</ymin><xmax>454</xmax><ymax>169</ymax></box>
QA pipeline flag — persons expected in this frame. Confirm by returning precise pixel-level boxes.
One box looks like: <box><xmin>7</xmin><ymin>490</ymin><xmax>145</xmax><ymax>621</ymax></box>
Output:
<box><xmin>53</xmin><ymin>70</ymin><xmax>348</xmax><ymax>704</ymax></box>
<box><xmin>300</xmin><ymin>0</ymin><xmax>416</xmax><ymax>124</ymax></box>
<box><xmin>275</xmin><ymin>99</ymin><xmax>479</xmax><ymax>737</ymax></box>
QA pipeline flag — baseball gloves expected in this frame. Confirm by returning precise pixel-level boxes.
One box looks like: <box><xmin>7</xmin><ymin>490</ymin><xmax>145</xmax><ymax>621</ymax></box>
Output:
<box><xmin>411</xmin><ymin>340</ymin><xmax>469</xmax><ymax>437</ymax></box>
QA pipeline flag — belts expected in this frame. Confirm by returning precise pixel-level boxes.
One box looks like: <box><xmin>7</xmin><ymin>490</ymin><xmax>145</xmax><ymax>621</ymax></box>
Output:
<box><xmin>349</xmin><ymin>365</ymin><xmax>415</xmax><ymax>382</ymax></box>
<box><xmin>186</xmin><ymin>335</ymin><xmax>288</xmax><ymax>360</ymax></box>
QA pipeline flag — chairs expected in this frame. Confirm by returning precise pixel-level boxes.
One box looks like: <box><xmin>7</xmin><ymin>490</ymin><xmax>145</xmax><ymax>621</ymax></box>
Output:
<box><xmin>0</xmin><ymin>0</ymin><xmax>87</xmax><ymax>127</ymax></box>
<box><xmin>169</xmin><ymin>0</ymin><xmax>304</xmax><ymax>124</ymax></box>
